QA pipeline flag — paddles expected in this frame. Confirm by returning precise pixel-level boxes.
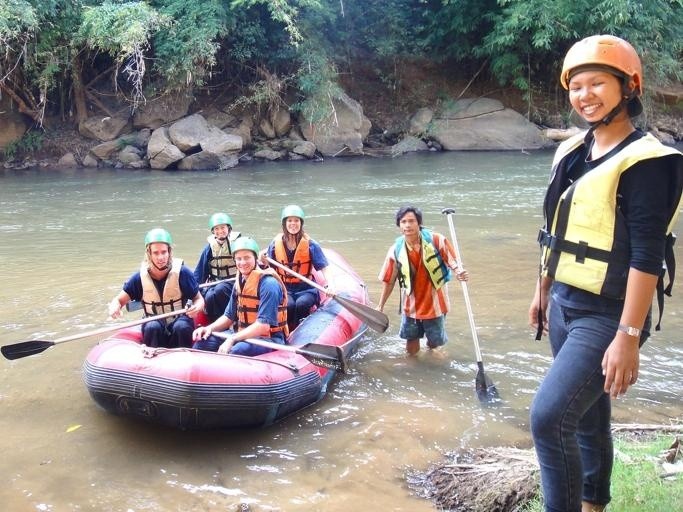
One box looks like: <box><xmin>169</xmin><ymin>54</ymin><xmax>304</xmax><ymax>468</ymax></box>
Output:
<box><xmin>442</xmin><ymin>208</ymin><xmax>500</xmax><ymax>402</ymax></box>
<box><xmin>126</xmin><ymin>277</ymin><xmax>237</xmax><ymax>311</ymax></box>
<box><xmin>212</xmin><ymin>332</ymin><xmax>346</xmax><ymax>374</ymax></box>
<box><xmin>266</xmin><ymin>257</ymin><xmax>389</xmax><ymax>333</ymax></box>
<box><xmin>1</xmin><ymin>307</ymin><xmax>195</xmax><ymax>360</ymax></box>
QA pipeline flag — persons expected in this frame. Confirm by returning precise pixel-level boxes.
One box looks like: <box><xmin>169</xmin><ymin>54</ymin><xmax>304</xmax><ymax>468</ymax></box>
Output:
<box><xmin>375</xmin><ymin>206</ymin><xmax>469</xmax><ymax>354</ymax></box>
<box><xmin>527</xmin><ymin>34</ymin><xmax>683</xmax><ymax>512</ymax></box>
<box><xmin>192</xmin><ymin>237</ymin><xmax>290</xmax><ymax>357</ymax></box>
<box><xmin>257</xmin><ymin>205</ymin><xmax>335</xmax><ymax>335</ymax></box>
<box><xmin>193</xmin><ymin>213</ymin><xmax>242</xmax><ymax>323</ymax></box>
<box><xmin>109</xmin><ymin>228</ymin><xmax>205</xmax><ymax>349</ymax></box>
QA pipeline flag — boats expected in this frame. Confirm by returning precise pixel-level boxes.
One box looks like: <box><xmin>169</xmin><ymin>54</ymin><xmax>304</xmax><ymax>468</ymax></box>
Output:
<box><xmin>79</xmin><ymin>248</ymin><xmax>376</xmax><ymax>433</ymax></box>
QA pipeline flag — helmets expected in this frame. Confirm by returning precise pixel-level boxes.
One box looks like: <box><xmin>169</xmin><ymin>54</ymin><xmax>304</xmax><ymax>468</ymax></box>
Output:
<box><xmin>145</xmin><ymin>205</ymin><xmax>304</xmax><ymax>259</ymax></box>
<box><xmin>560</xmin><ymin>34</ymin><xmax>643</xmax><ymax>97</ymax></box>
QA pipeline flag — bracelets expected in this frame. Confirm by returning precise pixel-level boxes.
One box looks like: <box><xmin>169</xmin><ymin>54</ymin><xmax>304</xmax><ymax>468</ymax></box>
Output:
<box><xmin>617</xmin><ymin>324</ymin><xmax>643</xmax><ymax>337</ymax></box>
